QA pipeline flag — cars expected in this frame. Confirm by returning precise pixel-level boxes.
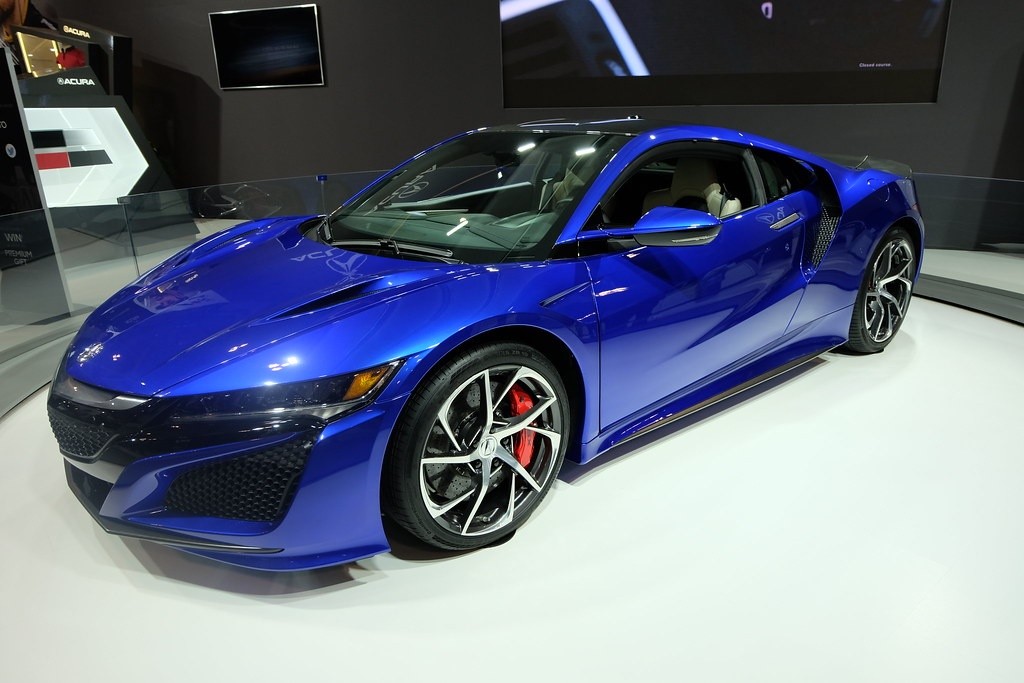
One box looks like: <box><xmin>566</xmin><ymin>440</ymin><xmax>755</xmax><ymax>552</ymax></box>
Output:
<box><xmin>46</xmin><ymin>117</ymin><xmax>925</xmax><ymax>575</ymax></box>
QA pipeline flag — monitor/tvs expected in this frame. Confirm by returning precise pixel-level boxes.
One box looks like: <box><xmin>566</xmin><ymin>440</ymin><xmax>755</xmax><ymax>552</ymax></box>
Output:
<box><xmin>208</xmin><ymin>3</ymin><xmax>325</xmax><ymax>90</ymax></box>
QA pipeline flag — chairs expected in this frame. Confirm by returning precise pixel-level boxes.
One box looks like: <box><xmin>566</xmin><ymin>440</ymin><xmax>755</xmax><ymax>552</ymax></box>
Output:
<box><xmin>641</xmin><ymin>156</ymin><xmax>719</xmax><ymax>215</ymax></box>
